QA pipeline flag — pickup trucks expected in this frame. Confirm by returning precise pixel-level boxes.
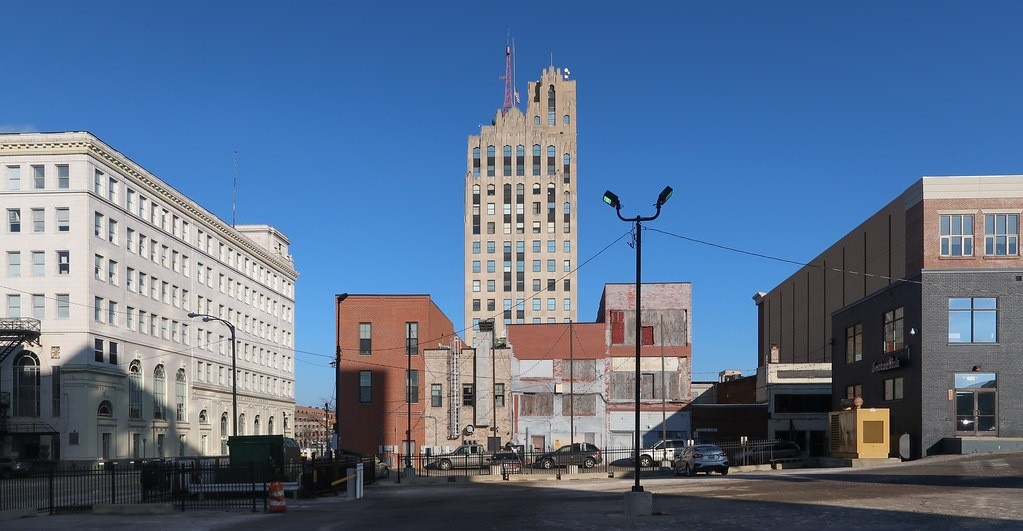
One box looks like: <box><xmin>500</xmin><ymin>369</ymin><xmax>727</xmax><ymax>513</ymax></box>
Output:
<box><xmin>634</xmin><ymin>438</ymin><xmax>686</xmax><ymax>468</ymax></box>
<box><xmin>435</xmin><ymin>444</ymin><xmax>493</xmax><ymax>470</ymax></box>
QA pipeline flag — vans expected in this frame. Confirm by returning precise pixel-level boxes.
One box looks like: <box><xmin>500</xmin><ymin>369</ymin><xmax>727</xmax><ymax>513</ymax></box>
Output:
<box><xmin>309</xmin><ymin>443</ymin><xmax>325</xmax><ymax>457</ymax></box>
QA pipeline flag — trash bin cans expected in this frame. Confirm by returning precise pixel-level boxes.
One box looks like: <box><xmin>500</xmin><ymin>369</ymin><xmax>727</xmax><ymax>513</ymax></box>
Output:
<box><xmin>228</xmin><ymin>435</ymin><xmax>303</xmax><ymax>496</ymax></box>
<box><xmin>142</xmin><ymin>462</ymin><xmax>171</xmax><ymax>490</ymax></box>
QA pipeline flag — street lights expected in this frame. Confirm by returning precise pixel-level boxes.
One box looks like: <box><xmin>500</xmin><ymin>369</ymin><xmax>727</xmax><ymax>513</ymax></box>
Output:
<box><xmin>601</xmin><ymin>186</ymin><xmax>674</xmax><ymax>492</ymax></box>
<box><xmin>187</xmin><ymin>312</ymin><xmax>237</xmax><ymax>436</ymax></box>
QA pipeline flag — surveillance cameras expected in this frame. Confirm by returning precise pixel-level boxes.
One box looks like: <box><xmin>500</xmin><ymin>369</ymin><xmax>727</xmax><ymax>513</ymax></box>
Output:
<box><xmin>909</xmin><ymin>329</ymin><xmax>916</xmax><ymax>335</ymax></box>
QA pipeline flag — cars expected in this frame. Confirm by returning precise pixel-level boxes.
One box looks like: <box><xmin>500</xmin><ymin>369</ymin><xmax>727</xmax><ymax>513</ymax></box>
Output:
<box><xmin>485</xmin><ymin>451</ymin><xmax>523</xmax><ymax>475</ymax></box>
<box><xmin>672</xmin><ymin>444</ymin><xmax>730</xmax><ymax>477</ymax></box>
<box><xmin>0</xmin><ymin>456</ymin><xmax>33</xmax><ymax>480</ymax></box>
<box><xmin>375</xmin><ymin>457</ymin><xmax>390</xmax><ymax>480</ymax></box>
<box><xmin>732</xmin><ymin>441</ymin><xmax>802</xmax><ymax>464</ymax></box>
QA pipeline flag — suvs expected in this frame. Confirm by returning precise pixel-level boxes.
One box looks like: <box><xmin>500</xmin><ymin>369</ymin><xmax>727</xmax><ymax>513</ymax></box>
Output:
<box><xmin>535</xmin><ymin>442</ymin><xmax>603</xmax><ymax>470</ymax></box>
